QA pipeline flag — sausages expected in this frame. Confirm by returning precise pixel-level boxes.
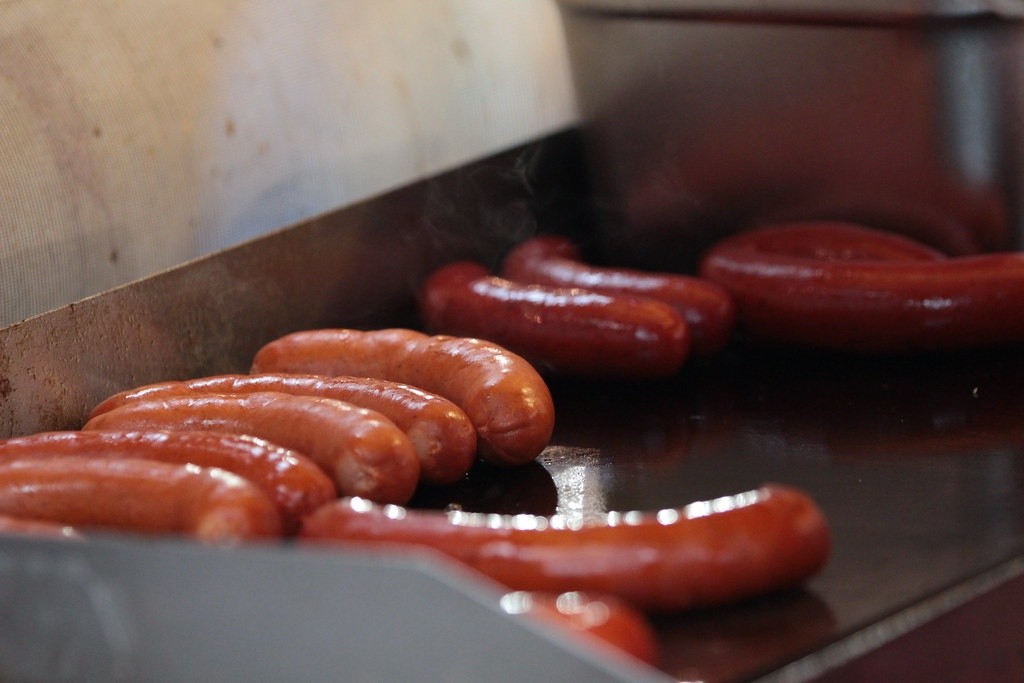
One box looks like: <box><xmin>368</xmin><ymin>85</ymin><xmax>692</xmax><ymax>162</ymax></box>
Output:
<box><xmin>0</xmin><ymin>219</ymin><xmax>1024</xmax><ymax>667</ymax></box>
